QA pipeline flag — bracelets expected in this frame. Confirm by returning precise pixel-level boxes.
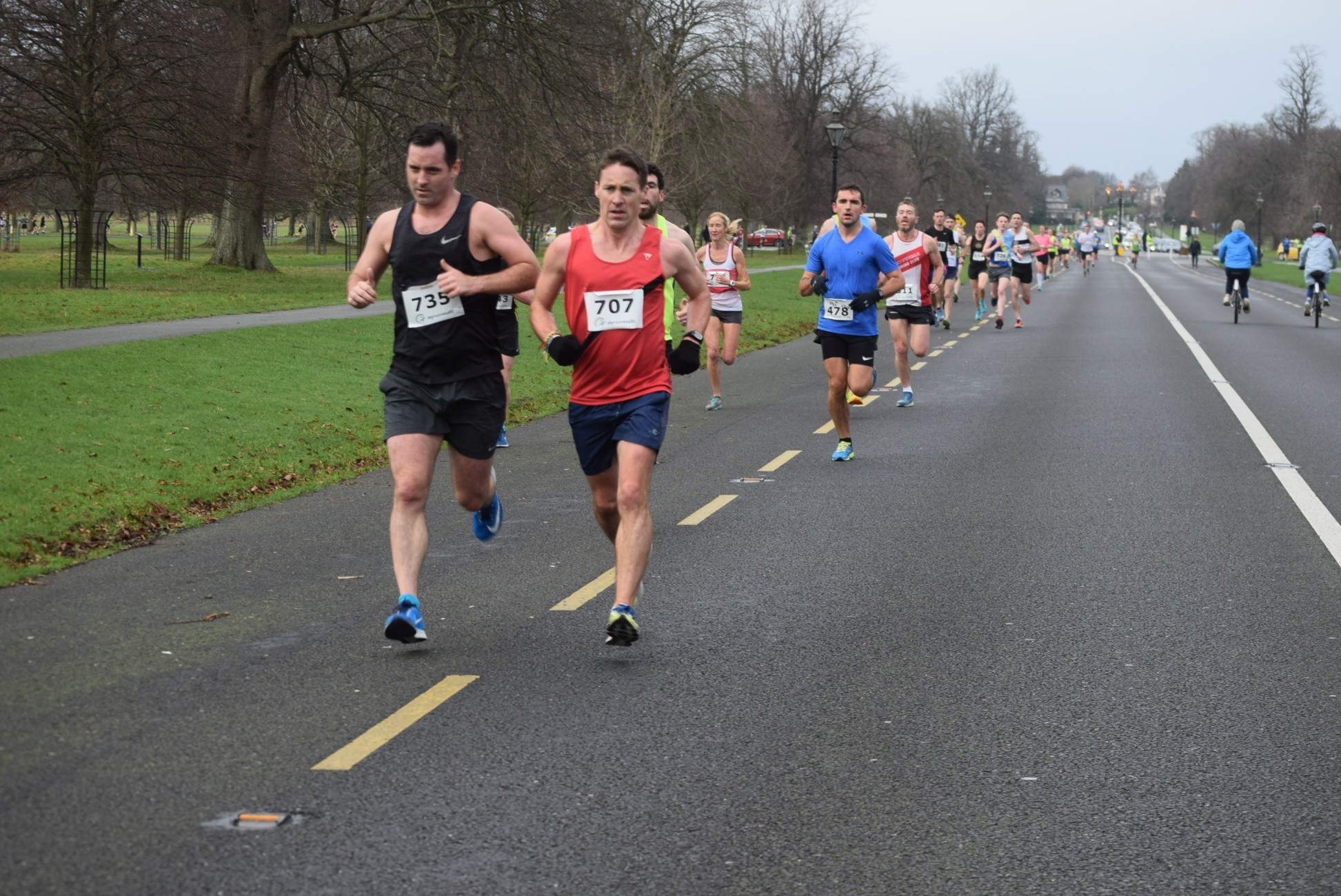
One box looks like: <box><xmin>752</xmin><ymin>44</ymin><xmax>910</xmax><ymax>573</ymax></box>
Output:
<box><xmin>961</xmin><ymin>255</ymin><xmax>965</xmax><ymax>260</ymax></box>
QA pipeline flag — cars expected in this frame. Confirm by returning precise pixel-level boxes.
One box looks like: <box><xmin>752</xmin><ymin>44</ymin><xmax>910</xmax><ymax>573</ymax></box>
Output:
<box><xmin>1107</xmin><ymin>219</ymin><xmax>1142</xmax><ymax>236</ymax></box>
<box><xmin>1212</xmin><ymin>241</ymin><xmax>1263</xmax><ymax>258</ymax></box>
<box><xmin>1079</xmin><ymin>217</ymin><xmax>1104</xmax><ymax>232</ymax></box>
<box><xmin>1277</xmin><ymin>238</ymin><xmax>1307</xmax><ymax>260</ymax></box>
<box><xmin>731</xmin><ymin>228</ymin><xmax>795</xmax><ymax>248</ymax></box>
<box><xmin>1150</xmin><ymin>237</ymin><xmax>1183</xmax><ymax>254</ymax></box>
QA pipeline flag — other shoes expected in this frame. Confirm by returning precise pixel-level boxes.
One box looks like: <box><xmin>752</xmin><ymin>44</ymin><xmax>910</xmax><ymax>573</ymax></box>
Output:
<box><xmin>1305</xmin><ymin>301</ymin><xmax>1311</xmax><ymax>316</ymax></box>
<box><xmin>934</xmin><ymin>262</ymin><xmax>1096</xmax><ymax>329</ymax></box>
<box><xmin>1223</xmin><ymin>298</ymin><xmax>1230</xmax><ymax>306</ymax></box>
<box><xmin>1243</xmin><ymin>300</ymin><xmax>1250</xmax><ymax>314</ymax></box>
<box><xmin>1324</xmin><ymin>298</ymin><xmax>1330</xmax><ymax>306</ymax></box>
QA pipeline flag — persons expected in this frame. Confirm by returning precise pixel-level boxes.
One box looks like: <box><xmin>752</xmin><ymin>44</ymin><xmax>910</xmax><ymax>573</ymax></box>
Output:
<box><xmin>524</xmin><ymin>220</ymin><xmax>577</xmax><ymax>244</ymax></box>
<box><xmin>1282</xmin><ymin>237</ymin><xmax>1304</xmax><ymax>260</ymax></box>
<box><xmin>1113</xmin><ymin>231</ymin><xmax>1154</xmax><ymax>268</ymax></box>
<box><xmin>366</xmin><ymin>216</ymin><xmax>373</xmax><ymax>234</ymax></box>
<box><xmin>878</xmin><ymin>200</ymin><xmax>944</xmax><ymax>408</ymax></box>
<box><xmin>1218</xmin><ymin>220</ymin><xmax>1259</xmax><ymax>312</ymax></box>
<box><xmin>263</xmin><ymin>217</ymin><xmax>306</xmax><ymax>238</ymax></box>
<box><xmin>922</xmin><ymin>209</ymin><xmax>967</xmax><ymax>326</ymax></box>
<box><xmin>1298</xmin><ymin>223</ymin><xmax>1339</xmax><ymax>316</ymax></box>
<box><xmin>72</xmin><ymin>218</ymin><xmax>78</xmax><ymax>234</ymax></box>
<box><xmin>105</xmin><ymin>214</ymin><xmax>112</xmax><ymax>236</ymax></box>
<box><xmin>737</xmin><ymin>224</ymin><xmax>820</xmax><ymax>248</ymax></box>
<box><xmin>798</xmin><ymin>185</ymin><xmax>905</xmax><ymax>461</ymax></box>
<box><xmin>529</xmin><ymin>145</ymin><xmax>712</xmax><ymax>646</ymax></box>
<box><xmin>346</xmin><ymin>123</ymin><xmax>539</xmax><ymax>643</ymax></box>
<box><xmin>0</xmin><ymin>212</ymin><xmax>47</xmax><ymax>236</ymax></box>
<box><xmin>1188</xmin><ymin>237</ymin><xmax>1201</xmax><ymax>269</ymax></box>
<box><xmin>331</xmin><ymin>220</ymin><xmax>338</xmax><ymax>239</ymax></box>
<box><xmin>696</xmin><ymin>211</ymin><xmax>751</xmax><ymax>412</ymax></box>
<box><xmin>958</xmin><ymin>212</ymin><xmax>1101</xmax><ymax>329</ymax></box>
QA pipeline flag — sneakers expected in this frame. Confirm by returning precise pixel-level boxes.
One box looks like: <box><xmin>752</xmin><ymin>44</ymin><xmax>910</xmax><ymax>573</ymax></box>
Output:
<box><xmin>383</xmin><ymin>599</ymin><xmax>428</xmax><ymax>643</ymax></box>
<box><xmin>705</xmin><ymin>396</ymin><xmax>722</xmax><ymax>411</ymax></box>
<box><xmin>606</xmin><ymin>605</ymin><xmax>639</xmax><ymax>648</ymax></box>
<box><xmin>830</xmin><ymin>440</ymin><xmax>856</xmax><ymax>462</ymax></box>
<box><xmin>496</xmin><ymin>428</ymin><xmax>509</xmax><ymax>447</ymax></box>
<box><xmin>473</xmin><ymin>492</ymin><xmax>502</xmax><ymax>542</ymax></box>
<box><xmin>872</xmin><ymin>366</ymin><xmax>878</xmax><ymax>386</ymax></box>
<box><xmin>847</xmin><ymin>389</ymin><xmax>862</xmax><ymax>405</ymax></box>
<box><xmin>897</xmin><ymin>394</ymin><xmax>914</xmax><ymax>408</ymax></box>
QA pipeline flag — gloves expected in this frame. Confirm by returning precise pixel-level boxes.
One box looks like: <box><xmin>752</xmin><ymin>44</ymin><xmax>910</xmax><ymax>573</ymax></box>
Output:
<box><xmin>811</xmin><ymin>276</ymin><xmax>829</xmax><ymax>296</ymax></box>
<box><xmin>548</xmin><ymin>334</ymin><xmax>582</xmax><ymax>366</ymax></box>
<box><xmin>849</xmin><ymin>290</ymin><xmax>880</xmax><ymax>312</ymax></box>
<box><xmin>669</xmin><ymin>340</ymin><xmax>702</xmax><ymax>376</ymax></box>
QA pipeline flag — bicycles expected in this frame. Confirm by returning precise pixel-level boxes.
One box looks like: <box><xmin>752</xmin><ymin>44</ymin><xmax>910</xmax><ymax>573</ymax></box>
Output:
<box><xmin>1299</xmin><ymin>266</ymin><xmax>1334</xmax><ymax>329</ymax></box>
<box><xmin>1224</xmin><ymin>259</ymin><xmax>1261</xmax><ymax>325</ymax></box>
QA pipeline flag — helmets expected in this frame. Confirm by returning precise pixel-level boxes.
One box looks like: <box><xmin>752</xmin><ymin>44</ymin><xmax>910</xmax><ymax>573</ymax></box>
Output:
<box><xmin>1313</xmin><ymin>222</ymin><xmax>1327</xmax><ymax>234</ymax></box>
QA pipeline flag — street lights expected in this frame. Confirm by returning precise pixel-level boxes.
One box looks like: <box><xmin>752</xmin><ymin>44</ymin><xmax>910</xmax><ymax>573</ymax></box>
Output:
<box><xmin>825</xmin><ymin>107</ymin><xmax>847</xmax><ymax>218</ymax></box>
<box><xmin>1312</xmin><ymin>200</ymin><xmax>1322</xmax><ymax>224</ymax></box>
<box><xmin>1105</xmin><ymin>180</ymin><xmax>1137</xmax><ymax>233</ymax></box>
<box><xmin>982</xmin><ymin>185</ymin><xmax>993</xmax><ymax>237</ymax></box>
<box><xmin>1255</xmin><ymin>191</ymin><xmax>1265</xmax><ymax>266</ymax></box>
<box><xmin>1028</xmin><ymin>207</ymin><xmax>1034</xmax><ymax>229</ymax></box>
<box><xmin>1172</xmin><ymin>216</ymin><xmax>1176</xmax><ymax>240</ymax></box>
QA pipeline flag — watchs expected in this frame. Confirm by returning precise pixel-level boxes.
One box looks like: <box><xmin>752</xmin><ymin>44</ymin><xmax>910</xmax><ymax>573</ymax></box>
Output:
<box><xmin>877</xmin><ymin>289</ymin><xmax>885</xmax><ymax>299</ymax></box>
<box><xmin>682</xmin><ymin>330</ymin><xmax>704</xmax><ymax>345</ymax></box>
<box><xmin>729</xmin><ymin>280</ymin><xmax>735</xmax><ymax>286</ymax></box>
